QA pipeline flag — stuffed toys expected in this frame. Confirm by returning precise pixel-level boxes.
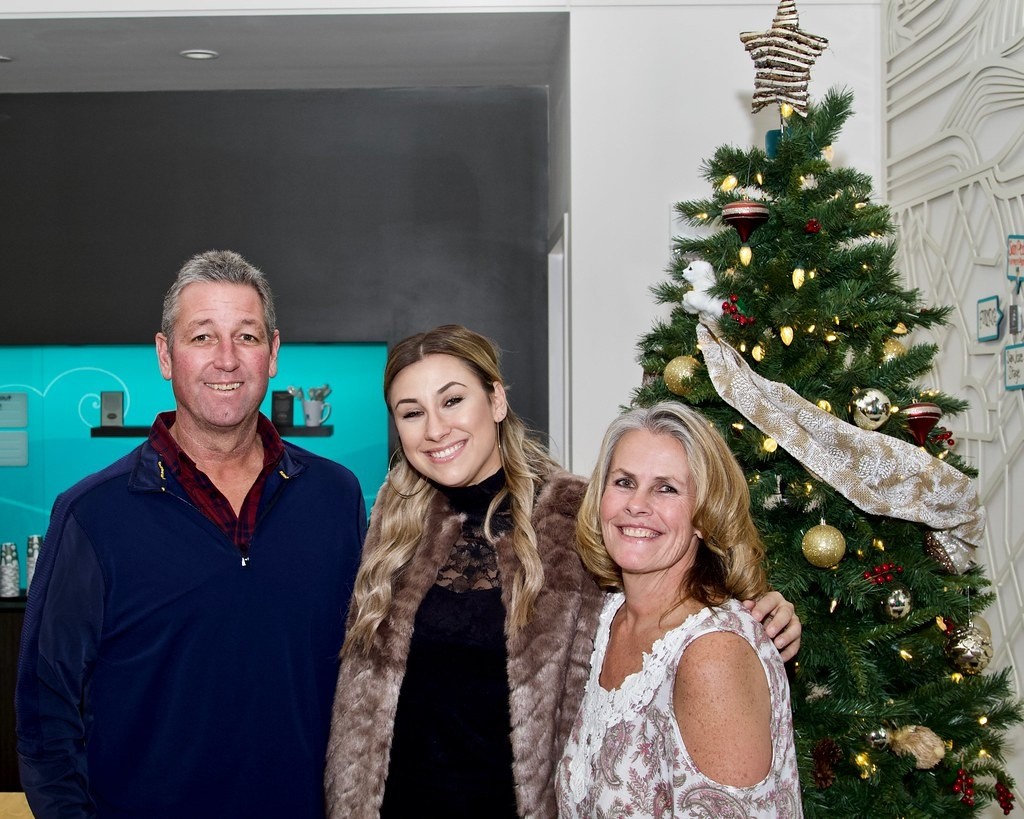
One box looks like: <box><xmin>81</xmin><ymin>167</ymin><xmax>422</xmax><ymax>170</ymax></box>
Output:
<box><xmin>682</xmin><ymin>260</ymin><xmax>726</xmax><ymax>317</ymax></box>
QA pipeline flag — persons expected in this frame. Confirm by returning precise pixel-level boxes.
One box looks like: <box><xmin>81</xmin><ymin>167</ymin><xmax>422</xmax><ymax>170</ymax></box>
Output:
<box><xmin>553</xmin><ymin>402</ymin><xmax>804</xmax><ymax>819</ymax></box>
<box><xmin>14</xmin><ymin>250</ymin><xmax>366</xmax><ymax>818</ymax></box>
<box><xmin>328</xmin><ymin>325</ymin><xmax>802</xmax><ymax>818</ymax></box>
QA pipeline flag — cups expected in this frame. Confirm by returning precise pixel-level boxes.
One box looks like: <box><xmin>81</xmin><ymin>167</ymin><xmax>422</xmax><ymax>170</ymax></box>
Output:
<box><xmin>0</xmin><ymin>543</ymin><xmax>21</xmax><ymax>597</ymax></box>
<box><xmin>26</xmin><ymin>535</ymin><xmax>43</xmax><ymax>597</ymax></box>
<box><xmin>304</xmin><ymin>400</ymin><xmax>331</xmax><ymax>427</ymax></box>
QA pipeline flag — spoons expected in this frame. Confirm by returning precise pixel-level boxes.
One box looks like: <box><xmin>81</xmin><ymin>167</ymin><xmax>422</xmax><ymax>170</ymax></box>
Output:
<box><xmin>288</xmin><ymin>384</ymin><xmax>332</xmax><ymax>401</ymax></box>
